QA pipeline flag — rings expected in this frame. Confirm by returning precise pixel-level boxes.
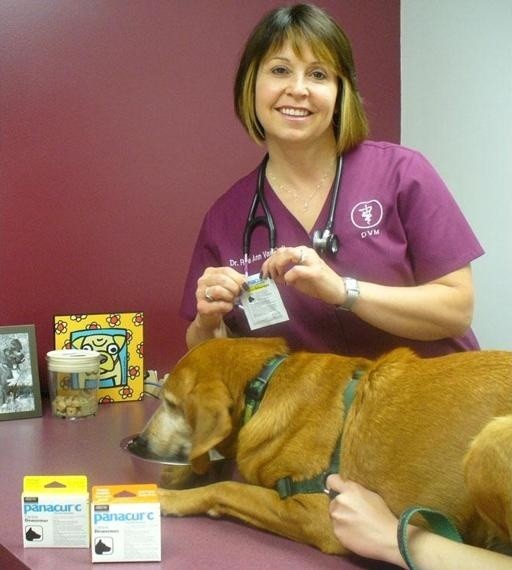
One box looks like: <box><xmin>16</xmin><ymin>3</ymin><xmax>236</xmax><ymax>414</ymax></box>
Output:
<box><xmin>297</xmin><ymin>248</ymin><xmax>307</xmax><ymax>263</ymax></box>
<box><xmin>204</xmin><ymin>287</ymin><xmax>215</xmax><ymax>302</ymax></box>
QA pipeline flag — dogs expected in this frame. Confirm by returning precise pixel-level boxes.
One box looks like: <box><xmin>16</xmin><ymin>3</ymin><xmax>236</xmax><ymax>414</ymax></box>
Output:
<box><xmin>124</xmin><ymin>335</ymin><xmax>511</xmax><ymax>569</ymax></box>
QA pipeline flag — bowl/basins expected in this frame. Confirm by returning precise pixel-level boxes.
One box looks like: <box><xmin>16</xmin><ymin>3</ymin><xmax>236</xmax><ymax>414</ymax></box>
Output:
<box><xmin>121</xmin><ymin>433</ymin><xmax>226</xmax><ymax>490</ymax></box>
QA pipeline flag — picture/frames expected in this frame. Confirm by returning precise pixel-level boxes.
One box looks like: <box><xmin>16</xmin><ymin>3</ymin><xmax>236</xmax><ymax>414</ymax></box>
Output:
<box><xmin>0</xmin><ymin>323</ymin><xmax>42</xmax><ymax>421</ymax></box>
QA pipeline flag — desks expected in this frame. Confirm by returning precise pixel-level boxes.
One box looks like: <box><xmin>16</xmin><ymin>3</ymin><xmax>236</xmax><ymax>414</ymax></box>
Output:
<box><xmin>1</xmin><ymin>394</ymin><xmax>409</xmax><ymax>570</ymax></box>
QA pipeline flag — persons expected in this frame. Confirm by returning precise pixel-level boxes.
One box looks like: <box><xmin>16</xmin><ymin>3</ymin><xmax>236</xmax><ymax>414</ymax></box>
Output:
<box><xmin>179</xmin><ymin>2</ymin><xmax>482</xmax><ymax>368</ymax></box>
<box><xmin>323</xmin><ymin>472</ymin><xmax>511</xmax><ymax>570</ymax></box>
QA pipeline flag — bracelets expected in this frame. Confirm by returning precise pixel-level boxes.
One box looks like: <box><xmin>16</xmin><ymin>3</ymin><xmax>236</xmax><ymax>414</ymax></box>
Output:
<box><xmin>335</xmin><ymin>276</ymin><xmax>363</xmax><ymax>313</ymax></box>
<box><xmin>395</xmin><ymin>505</ymin><xmax>464</xmax><ymax>570</ymax></box>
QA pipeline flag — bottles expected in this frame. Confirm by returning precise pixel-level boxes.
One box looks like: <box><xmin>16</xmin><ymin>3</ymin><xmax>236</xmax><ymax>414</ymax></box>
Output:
<box><xmin>44</xmin><ymin>350</ymin><xmax>101</xmax><ymax>418</ymax></box>
